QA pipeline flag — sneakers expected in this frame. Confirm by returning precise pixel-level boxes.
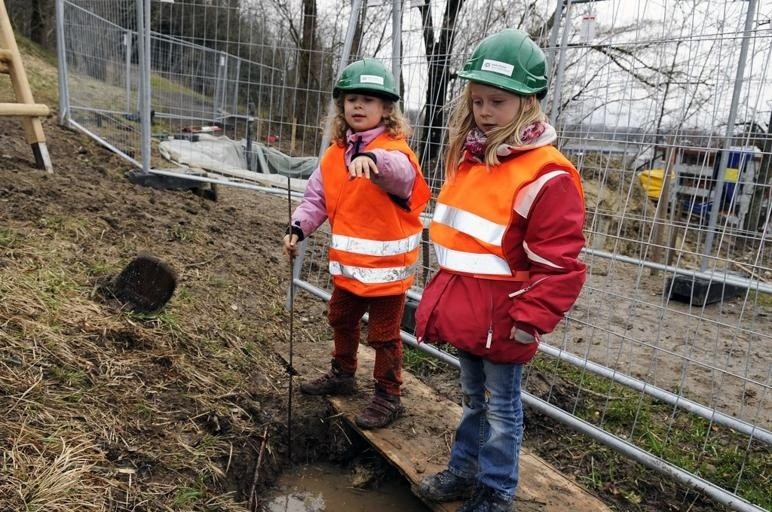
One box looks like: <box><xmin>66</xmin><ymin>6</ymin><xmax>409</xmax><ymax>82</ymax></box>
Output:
<box><xmin>300</xmin><ymin>360</ymin><xmax>357</xmax><ymax>396</ymax></box>
<box><xmin>455</xmin><ymin>482</ymin><xmax>513</xmax><ymax>512</ymax></box>
<box><xmin>354</xmin><ymin>392</ymin><xmax>402</xmax><ymax>430</ymax></box>
<box><xmin>419</xmin><ymin>470</ymin><xmax>475</xmax><ymax>503</ymax></box>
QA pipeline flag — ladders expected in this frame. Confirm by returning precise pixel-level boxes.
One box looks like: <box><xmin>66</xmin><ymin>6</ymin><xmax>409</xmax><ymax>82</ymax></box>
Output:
<box><xmin>0</xmin><ymin>0</ymin><xmax>54</xmax><ymax>175</ymax></box>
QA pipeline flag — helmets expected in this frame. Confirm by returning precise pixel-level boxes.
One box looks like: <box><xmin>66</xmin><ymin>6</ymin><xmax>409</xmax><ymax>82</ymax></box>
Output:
<box><xmin>333</xmin><ymin>59</ymin><xmax>400</xmax><ymax>104</ymax></box>
<box><xmin>457</xmin><ymin>29</ymin><xmax>549</xmax><ymax>101</ymax></box>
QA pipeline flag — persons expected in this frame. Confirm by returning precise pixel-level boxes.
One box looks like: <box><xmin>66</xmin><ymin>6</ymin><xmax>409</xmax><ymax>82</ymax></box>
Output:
<box><xmin>412</xmin><ymin>30</ymin><xmax>587</xmax><ymax>511</ymax></box>
<box><xmin>281</xmin><ymin>57</ymin><xmax>432</xmax><ymax>432</ymax></box>
